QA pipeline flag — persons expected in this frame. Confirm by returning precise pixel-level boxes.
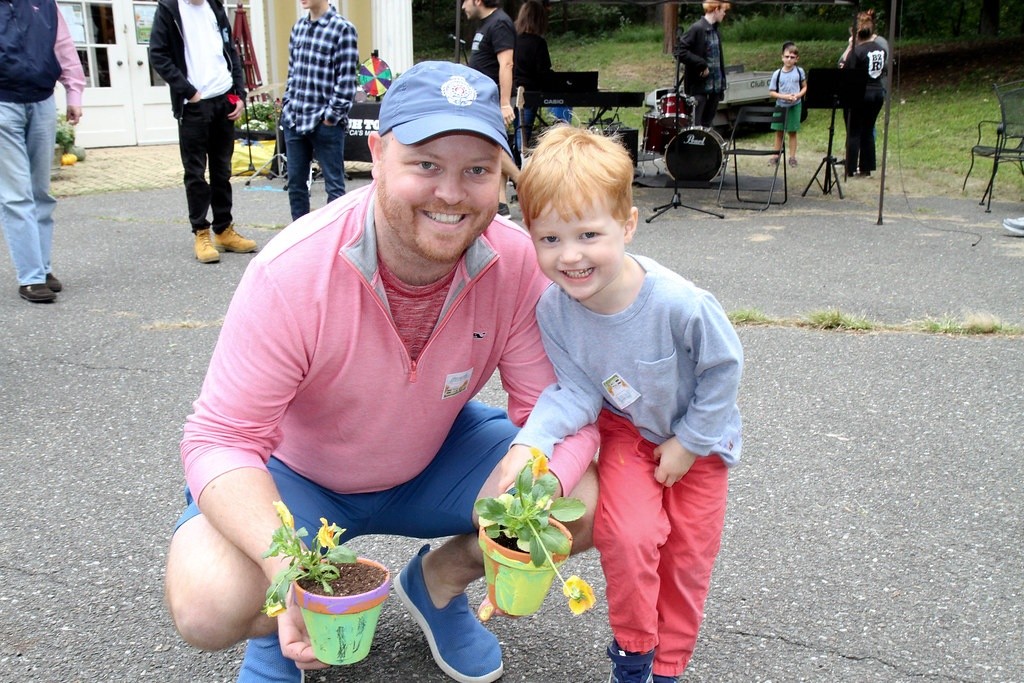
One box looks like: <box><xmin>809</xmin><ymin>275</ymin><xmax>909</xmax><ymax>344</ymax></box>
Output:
<box><xmin>461</xmin><ymin>0</ymin><xmax>557</xmax><ymax>222</ymax></box>
<box><xmin>0</xmin><ymin>0</ymin><xmax>87</xmax><ymax>302</ymax></box>
<box><xmin>162</xmin><ymin>61</ymin><xmax>609</xmax><ymax>683</ymax></box>
<box><xmin>279</xmin><ymin>0</ymin><xmax>357</xmax><ymax>224</ymax></box>
<box><xmin>765</xmin><ymin>43</ymin><xmax>810</xmax><ymax>168</ymax></box>
<box><xmin>150</xmin><ymin>0</ymin><xmax>258</xmax><ymax>262</ymax></box>
<box><xmin>498</xmin><ymin>125</ymin><xmax>745</xmax><ymax>683</ymax></box>
<box><xmin>840</xmin><ymin>8</ymin><xmax>890</xmax><ymax>179</ymax></box>
<box><xmin>673</xmin><ymin>0</ymin><xmax>732</xmax><ymax>126</ymax></box>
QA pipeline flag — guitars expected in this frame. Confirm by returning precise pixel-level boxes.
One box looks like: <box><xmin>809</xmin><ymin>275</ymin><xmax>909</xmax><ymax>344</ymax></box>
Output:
<box><xmin>513</xmin><ymin>87</ymin><xmax>533</xmax><ymax>191</ymax></box>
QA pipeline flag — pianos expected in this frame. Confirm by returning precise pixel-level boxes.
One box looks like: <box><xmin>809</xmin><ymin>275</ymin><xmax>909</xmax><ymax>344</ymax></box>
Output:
<box><xmin>511</xmin><ymin>90</ymin><xmax>646</xmax><ymax>147</ymax></box>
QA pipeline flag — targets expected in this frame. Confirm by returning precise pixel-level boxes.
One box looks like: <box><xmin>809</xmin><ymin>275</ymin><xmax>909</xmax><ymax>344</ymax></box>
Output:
<box><xmin>354</xmin><ymin>54</ymin><xmax>395</xmax><ymax>97</ymax></box>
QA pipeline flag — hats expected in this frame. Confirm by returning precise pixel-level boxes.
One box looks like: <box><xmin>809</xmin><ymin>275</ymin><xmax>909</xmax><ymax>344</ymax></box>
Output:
<box><xmin>379</xmin><ymin>61</ymin><xmax>513</xmax><ymax>161</ymax></box>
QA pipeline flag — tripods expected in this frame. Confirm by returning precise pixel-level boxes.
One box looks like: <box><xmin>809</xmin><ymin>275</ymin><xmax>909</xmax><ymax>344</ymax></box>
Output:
<box><xmin>802</xmin><ymin>67</ymin><xmax>867</xmax><ymax>199</ymax></box>
<box><xmin>245</xmin><ymin>108</ymin><xmax>288</xmax><ymax>186</ymax></box>
<box><xmin>232</xmin><ymin>67</ymin><xmax>271</xmax><ymax>178</ymax></box>
<box><xmin>646</xmin><ymin>38</ymin><xmax>725</xmax><ymax>222</ymax></box>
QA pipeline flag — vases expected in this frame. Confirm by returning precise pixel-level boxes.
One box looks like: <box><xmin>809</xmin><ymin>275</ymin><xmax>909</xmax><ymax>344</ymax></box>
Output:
<box><xmin>293</xmin><ymin>557</ymin><xmax>390</xmax><ymax>666</ymax></box>
<box><xmin>478</xmin><ymin>518</ymin><xmax>572</xmax><ymax>617</ymax></box>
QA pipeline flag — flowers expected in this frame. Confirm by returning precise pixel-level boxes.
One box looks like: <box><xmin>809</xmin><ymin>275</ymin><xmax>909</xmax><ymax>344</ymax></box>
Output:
<box><xmin>261</xmin><ymin>501</ymin><xmax>354</xmax><ymax>617</ymax></box>
<box><xmin>468</xmin><ymin>445</ymin><xmax>603</xmax><ymax>615</ymax></box>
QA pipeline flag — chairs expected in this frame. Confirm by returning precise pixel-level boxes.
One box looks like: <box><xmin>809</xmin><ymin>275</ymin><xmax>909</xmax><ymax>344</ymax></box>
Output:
<box><xmin>716</xmin><ymin>104</ymin><xmax>788</xmax><ymax>211</ymax></box>
<box><xmin>962</xmin><ymin>79</ymin><xmax>1024</xmax><ymax>212</ymax></box>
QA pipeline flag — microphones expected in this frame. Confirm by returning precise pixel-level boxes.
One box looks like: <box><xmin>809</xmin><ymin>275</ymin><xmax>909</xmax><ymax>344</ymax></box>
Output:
<box><xmin>448</xmin><ymin>34</ymin><xmax>466</xmax><ymax>44</ymax></box>
<box><xmin>677</xmin><ymin>27</ymin><xmax>684</xmax><ymax>38</ymax></box>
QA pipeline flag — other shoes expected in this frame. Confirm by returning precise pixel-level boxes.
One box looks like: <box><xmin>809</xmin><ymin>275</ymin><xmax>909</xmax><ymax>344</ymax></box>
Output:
<box><xmin>861</xmin><ymin>170</ymin><xmax>871</xmax><ymax>177</ymax></box>
<box><xmin>789</xmin><ymin>158</ymin><xmax>796</xmax><ymax>167</ymax></box>
<box><xmin>237</xmin><ymin>631</ymin><xmax>305</xmax><ymax>683</ymax></box>
<box><xmin>45</xmin><ymin>274</ymin><xmax>61</xmax><ymax>292</ymax></box>
<box><xmin>394</xmin><ymin>544</ymin><xmax>505</xmax><ymax>683</ymax></box>
<box><xmin>497</xmin><ymin>202</ymin><xmax>511</xmax><ymax>219</ymax></box>
<box><xmin>18</xmin><ymin>284</ymin><xmax>55</xmax><ymax>300</ymax></box>
<box><xmin>769</xmin><ymin>158</ymin><xmax>777</xmax><ymax>166</ymax></box>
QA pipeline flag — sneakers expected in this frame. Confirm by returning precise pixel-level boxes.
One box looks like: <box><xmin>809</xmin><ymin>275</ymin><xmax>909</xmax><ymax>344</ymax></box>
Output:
<box><xmin>1003</xmin><ymin>216</ymin><xmax>1024</xmax><ymax>236</ymax></box>
<box><xmin>606</xmin><ymin>635</ymin><xmax>678</xmax><ymax>683</ymax></box>
<box><xmin>215</xmin><ymin>225</ymin><xmax>258</xmax><ymax>253</ymax></box>
<box><xmin>194</xmin><ymin>229</ymin><xmax>220</xmax><ymax>263</ymax></box>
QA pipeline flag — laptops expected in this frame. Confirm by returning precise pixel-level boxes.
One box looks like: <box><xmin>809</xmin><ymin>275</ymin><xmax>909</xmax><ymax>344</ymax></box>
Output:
<box><xmin>551</xmin><ymin>72</ymin><xmax>598</xmax><ymax>94</ymax></box>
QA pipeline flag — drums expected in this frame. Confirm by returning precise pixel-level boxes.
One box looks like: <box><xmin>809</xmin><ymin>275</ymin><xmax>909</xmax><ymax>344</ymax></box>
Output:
<box><xmin>640</xmin><ymin>111</ymin><xmax>675</xmax><ymax>151</ymax></box>
<box><xmin>663</xmin><ymin>125</ymin><xmax>727</xmax><ymax>186</ymax></box>
<box><xmin>657</xmin><ymin>92</ymin><xmax>693</xmax><ymax>124</ymax></box>
